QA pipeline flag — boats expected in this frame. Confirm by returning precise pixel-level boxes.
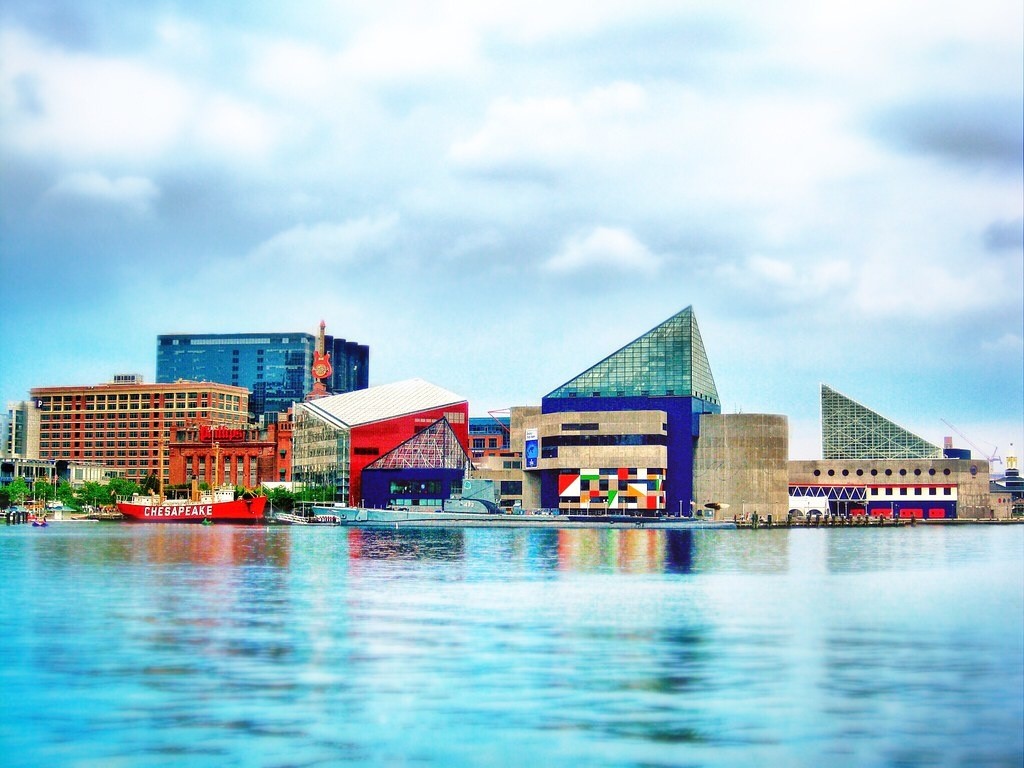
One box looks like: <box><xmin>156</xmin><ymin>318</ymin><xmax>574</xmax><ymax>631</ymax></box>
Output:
<box><xmin>32</xmin><ymin>521</ymin><xmax>49</xmax><ymax>527</ymax></box>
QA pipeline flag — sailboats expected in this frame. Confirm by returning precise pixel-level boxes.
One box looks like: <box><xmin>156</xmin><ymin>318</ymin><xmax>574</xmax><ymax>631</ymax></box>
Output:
<box><xmin>116</xmin><ymin>440</ymin><xmax>268</xmax><ymax>524</ymax></box>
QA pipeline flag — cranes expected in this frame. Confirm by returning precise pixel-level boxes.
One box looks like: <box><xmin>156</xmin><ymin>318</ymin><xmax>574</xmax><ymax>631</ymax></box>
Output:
<box><xmin>941</xmin><ymin>416</ymin><xmax>1002</xmax><ymax>466</ymax></box>
